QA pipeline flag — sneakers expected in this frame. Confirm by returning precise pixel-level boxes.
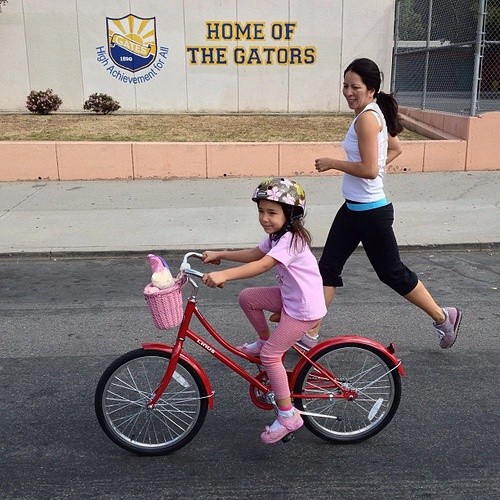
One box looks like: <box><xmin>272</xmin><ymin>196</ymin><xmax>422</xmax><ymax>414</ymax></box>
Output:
<box><xmin>295</xmin><ymin>333</ymin><xmax>319</xmax><ymax>351</ymax></box>
<box><xmin>433</xmin><ymin>306</ymin><xmax>463</xmax><ymax>349</ymax></box>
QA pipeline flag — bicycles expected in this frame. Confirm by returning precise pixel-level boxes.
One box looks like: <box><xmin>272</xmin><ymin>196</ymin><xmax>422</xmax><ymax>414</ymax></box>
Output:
<box><xmin>94</xmin><ymin>251</ymin><xmax>405</xmax><ymax>457</ymax></box>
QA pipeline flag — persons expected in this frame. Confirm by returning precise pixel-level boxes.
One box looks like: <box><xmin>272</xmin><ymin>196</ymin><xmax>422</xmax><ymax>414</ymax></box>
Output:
<box><xmin>291</xmin><ymin>58</ymin><xmax>464</xmax><ymax>352</ymax></box>
<box><xmin>200</xmin><ymin>176</ymin><xmax>327</xmax><ymax>444</ymax></box>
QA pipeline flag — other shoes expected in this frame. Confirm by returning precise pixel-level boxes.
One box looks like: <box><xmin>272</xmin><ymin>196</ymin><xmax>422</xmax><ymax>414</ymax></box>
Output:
<box><xmin>236</xmin><ymin>339</ymin><xmax>267</xmax><ymax>356</ymax></box>
<box><xmin>260</xmin><ymin>407</ymin><xmax>304</xmax><ymax>443</ymax></box>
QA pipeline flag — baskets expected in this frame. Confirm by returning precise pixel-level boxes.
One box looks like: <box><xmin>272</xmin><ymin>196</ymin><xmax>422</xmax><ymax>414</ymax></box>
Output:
<box><xmin>143</xmin><ymin>278</ymin><xmax>183</xmax><ymax>330</ymax></box>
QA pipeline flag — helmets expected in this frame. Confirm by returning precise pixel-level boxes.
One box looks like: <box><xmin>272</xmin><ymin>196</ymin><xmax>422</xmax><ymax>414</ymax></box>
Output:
<box><xmin>251</xmin><ymin>177</ymin><xmax>307</xmax><ymax>220</ymax></box>
